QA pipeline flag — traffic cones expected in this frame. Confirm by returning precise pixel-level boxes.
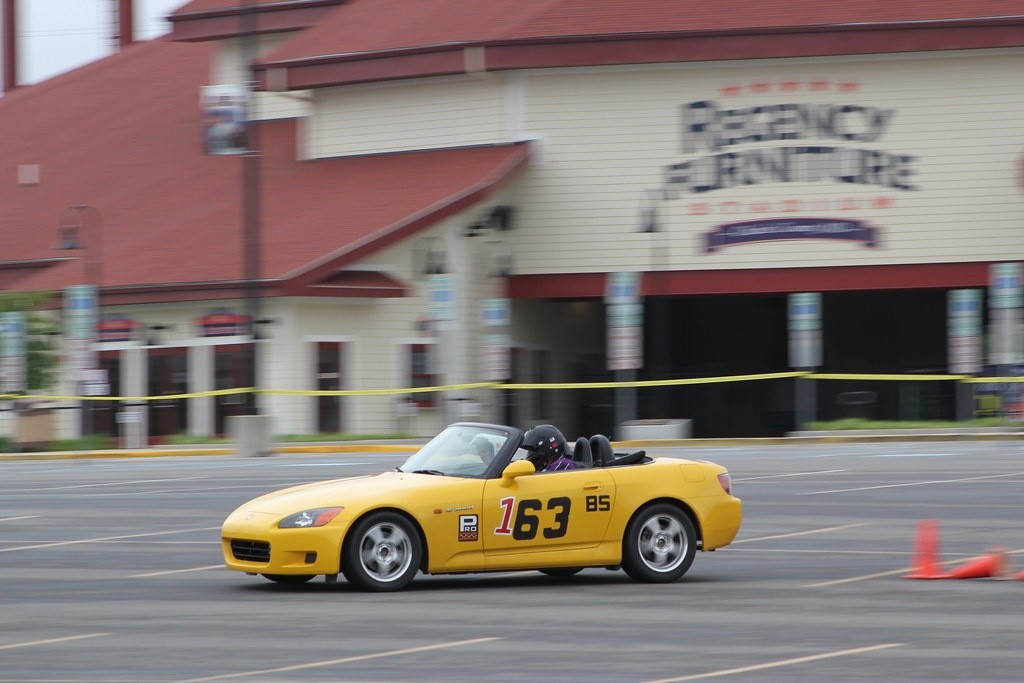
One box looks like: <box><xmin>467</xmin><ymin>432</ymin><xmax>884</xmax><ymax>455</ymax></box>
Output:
<box><xmin>901</xmin><ymin>519</ymin><xmax>953</xmax><ymax>579</ymax></box>
<box><xmin>946</xmin><ymin>550</ymin><xmax>1005</xmax><ymax>580</ymax></box>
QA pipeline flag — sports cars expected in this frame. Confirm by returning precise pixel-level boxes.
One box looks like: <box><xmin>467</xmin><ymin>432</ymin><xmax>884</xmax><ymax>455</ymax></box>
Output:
<box><xmin>218</xmin><ymin>418</ymin><xmax>745</xmax><ymax>594</ymax></box>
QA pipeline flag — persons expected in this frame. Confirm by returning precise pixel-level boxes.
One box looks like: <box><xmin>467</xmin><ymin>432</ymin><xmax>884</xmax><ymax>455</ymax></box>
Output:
<box><xmin>476</xmin><ymin>425</ymin><xmax>575</xmax><ymax>476</ymax></box>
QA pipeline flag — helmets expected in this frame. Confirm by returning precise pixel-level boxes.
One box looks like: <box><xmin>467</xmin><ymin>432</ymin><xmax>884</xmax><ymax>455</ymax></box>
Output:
<box><xmin>519</xmin><ymin>424</ymin><xmax>566</xmax><ymax>472</ymax></box>
